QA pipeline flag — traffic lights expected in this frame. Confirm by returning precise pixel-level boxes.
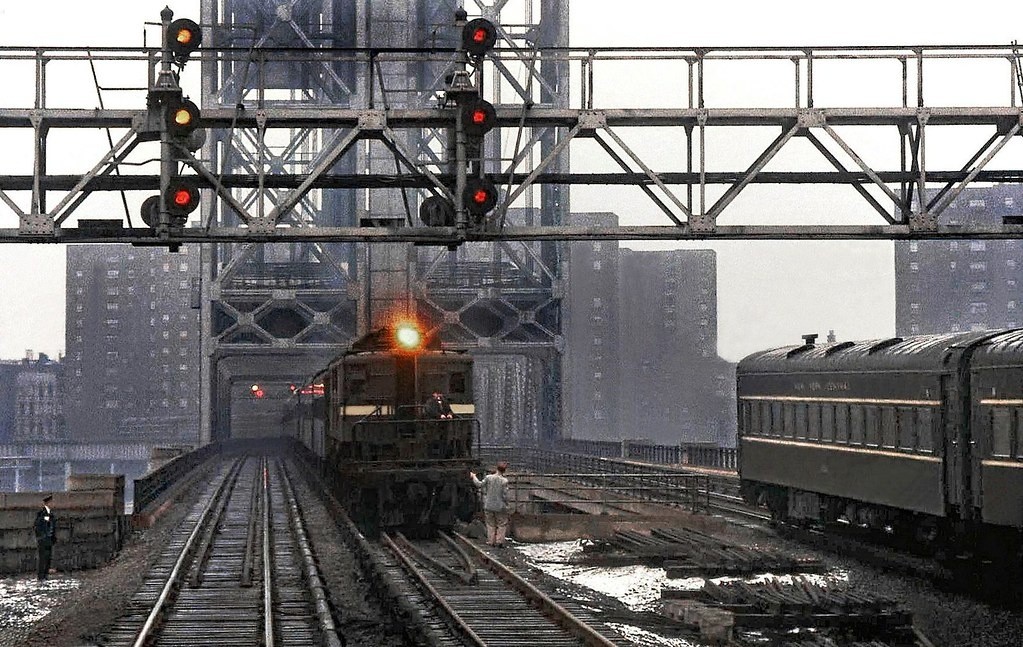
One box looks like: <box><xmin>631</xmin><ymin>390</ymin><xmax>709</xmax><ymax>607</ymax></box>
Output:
<box><xmin>461</xmin><ymin>18</ymin><xmax>497</xmax><ymax>60</ymax></box>
<box><xmin>165</xmin><ymin>18</ymin><xmax>203</xmax><ymax>55</ymax></box>
<box><xmin>165</xmin><ymin>99</ymin><xmax>202</xmax><ymax>137</ymax></box>
<box><xmin>463</xmin><ymin>178</ymin><xmax>497</xmax><ymax>215</ymax></box>
<box><xmin>461</xmin><ymin>99</ymin><xmax>496</xmax><ymax>136</ymax></box>
<box><xmin>164</xmin><ymin>180</ymin><xmax>200</xmax><ymax>216</ymax></box>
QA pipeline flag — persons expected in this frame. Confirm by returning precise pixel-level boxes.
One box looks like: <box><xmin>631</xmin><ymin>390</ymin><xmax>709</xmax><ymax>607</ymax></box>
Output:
<box><xmin>471</xmin><ymin>461</ymin><xmax>511</xmax><ymax>547</ymax></box>
<box><xmin>423</xmin><ymin>386</ymin><xmax>456</xmax><ymax>468</ymax></box>
<box><xmin>33</xmin><ymin>495</ymin><xmax>57</xmax><ymax>582</ymax></box>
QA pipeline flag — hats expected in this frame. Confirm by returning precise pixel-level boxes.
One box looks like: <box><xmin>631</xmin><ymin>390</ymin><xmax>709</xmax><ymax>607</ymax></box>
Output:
<box><xmin>497</xmin><ymin>463</ymin><xmax>507</xmax><ymax>472</ymax></box>
<box><xmin>43</xmin><ymin>495</ymin><xmax>54</xmax><ymax>503</ymax></box>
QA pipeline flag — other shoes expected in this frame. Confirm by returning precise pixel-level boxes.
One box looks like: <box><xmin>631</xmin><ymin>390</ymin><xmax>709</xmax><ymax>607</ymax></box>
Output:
<box><xmin>485</xmin><ymin>541</ymin><xmax>505</xmax><ymax>549</ymax></box>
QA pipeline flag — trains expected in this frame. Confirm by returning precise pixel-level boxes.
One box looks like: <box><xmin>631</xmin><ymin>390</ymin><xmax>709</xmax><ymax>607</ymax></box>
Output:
<box><xmin>282</xmin><ymin>321</ymin><xmax>482</xmax><ymax>540</ymax></box>
<box><xmin>736</xmin><ymin>327</ymin><xmax>1023</xmax><ymax>568</ymax></box>
